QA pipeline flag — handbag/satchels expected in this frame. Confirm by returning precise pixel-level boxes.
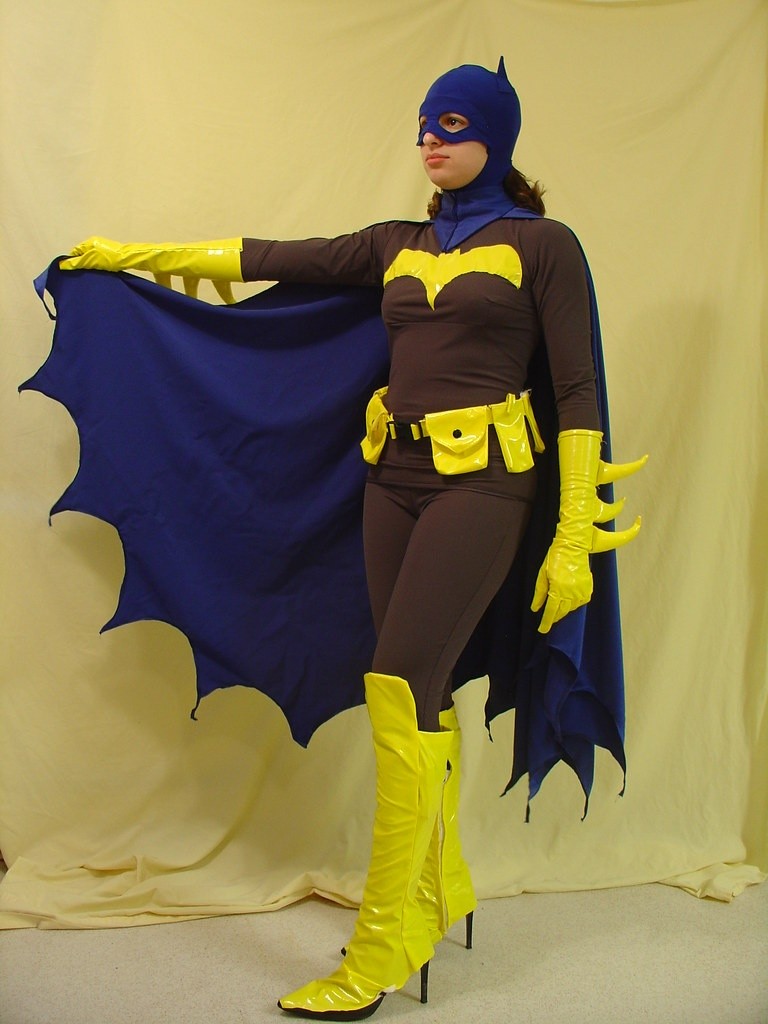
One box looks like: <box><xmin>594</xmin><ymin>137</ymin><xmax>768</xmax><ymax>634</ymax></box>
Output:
<box><xmin>361</xmin><ymin>386</ymin><xmax>545</xmax><ymax>475</ymax></box>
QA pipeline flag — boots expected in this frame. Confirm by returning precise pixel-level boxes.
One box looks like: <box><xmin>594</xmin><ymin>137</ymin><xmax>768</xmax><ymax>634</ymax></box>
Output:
<box><xmin>279</xmin><ymin>672</ymin><xmax>455</xmax><ymax>1020</ymax></box>
<box><xmin>342</xmin><ymin>700</ymin><xmax>477</xmax><ymax>956</ymax></box>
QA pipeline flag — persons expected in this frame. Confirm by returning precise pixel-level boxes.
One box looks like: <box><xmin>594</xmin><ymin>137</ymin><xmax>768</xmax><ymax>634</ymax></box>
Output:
<box><xmin>15</xmin><ymin>57</ymin><xmax>649</xmax><ymax>1021</ymax></box>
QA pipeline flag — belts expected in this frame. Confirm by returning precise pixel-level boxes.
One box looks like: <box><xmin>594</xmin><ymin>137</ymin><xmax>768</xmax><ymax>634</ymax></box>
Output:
<box><xmin>387</xmin><ymin>419</ymin><xmax>426</xmax><ymax>440</ymax></box>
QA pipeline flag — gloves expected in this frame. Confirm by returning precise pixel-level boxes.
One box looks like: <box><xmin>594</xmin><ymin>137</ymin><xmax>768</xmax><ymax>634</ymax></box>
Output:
<box><xmin>58</xmin><ymin>237</ymin><xmax>243</xmax><ymax>283</ymax></box>
<box><xmin>532</xmin><ymin>430</ymin><xmax>602</xmax><ymax>633</ymax></box>
